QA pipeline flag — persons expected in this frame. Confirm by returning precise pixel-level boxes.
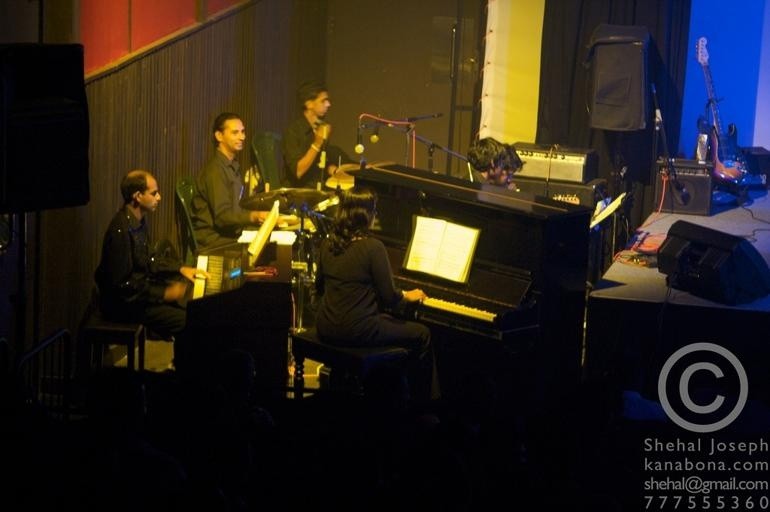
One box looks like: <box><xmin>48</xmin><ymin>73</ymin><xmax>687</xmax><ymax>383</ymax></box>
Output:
<box><xmin>192</xmin><ymin>112</ymin><xmax>301</xmax><ymax>282</ymax></box>
<box><xmin>464</xmin><ymin>137</ymin><xmax>506</xmax><ymax>186</ymax></box>
<box><xmin>1</xmin><ymin>335</ymin><xmax>496</xmax><ymax>510</ymax></box>
<box><xmin>92</xmin><ymin>169</ymin><xmax>210</xmax><ymax>338</ymax></box>
<box><xmin>496</xmin><ymin>143</ymin><xmax>524</xmax><ymax>192</ymax></box>
<box><xmin>276</xmin><ymin>83</ymin><xmax>359</xmax><ymax>189</ymax></box>
<box><xmin>315</xmin><ymin>186</ymin><xmax>444</xmax><ymax>426</ymax></box>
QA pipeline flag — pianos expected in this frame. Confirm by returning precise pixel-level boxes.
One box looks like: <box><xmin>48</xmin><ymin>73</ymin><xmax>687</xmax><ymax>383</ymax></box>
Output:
<box><xmin>176</xmin><ymin>239</ymin><xmax>291</xmax><ymax>390</ymax></box>
<box><xmin>311</xmin><ymin>163</ymin><xmax>589</xmax><ymax>420</ymax></box>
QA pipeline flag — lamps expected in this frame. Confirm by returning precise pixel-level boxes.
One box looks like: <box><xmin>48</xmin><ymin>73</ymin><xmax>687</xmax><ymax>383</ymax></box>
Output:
<box><xmin>352</xmin><ymin>112</ymin><xmax>416</xmax><ymax>170</ymax></box>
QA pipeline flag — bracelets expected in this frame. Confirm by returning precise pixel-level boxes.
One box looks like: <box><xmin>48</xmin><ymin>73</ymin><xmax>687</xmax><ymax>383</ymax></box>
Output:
<box><xmin>309</xmin><ymin>144</ymin><xmax>320</xmax><ymax>152</ymax></box>
<box><xmin>334</xmin><ymin>166</ymin><xmax>338</xmax><ymax>177</ymax></box>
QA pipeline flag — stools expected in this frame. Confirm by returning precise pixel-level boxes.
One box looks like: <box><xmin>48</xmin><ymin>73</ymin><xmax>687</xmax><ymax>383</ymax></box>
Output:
<box><xmin>81</xmin><ymin>318</ymin><xmax>146</xmax><ymax>378</ymax></box>
<box><xmin>286</xmin><ymin>320</ymin><xmax>417</xmax><ymax>406</ymax></box>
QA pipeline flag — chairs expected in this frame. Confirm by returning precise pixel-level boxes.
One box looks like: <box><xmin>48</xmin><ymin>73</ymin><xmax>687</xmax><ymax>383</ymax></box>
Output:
<box><xmin>249</xmin><ymin>129</ymin><xmax>292</xmax><ymax>192</ymax></box>
<box><xmin>172</xmin><ymin>174</ymin><xmax>243</xmax><ymax>269</ymax></box>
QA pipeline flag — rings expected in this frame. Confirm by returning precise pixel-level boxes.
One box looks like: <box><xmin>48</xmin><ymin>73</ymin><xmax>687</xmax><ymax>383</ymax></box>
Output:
<box><xmin>511</xmin><ymin>185</ymin><xmax>514</xmax><ymax>189</ymax></box>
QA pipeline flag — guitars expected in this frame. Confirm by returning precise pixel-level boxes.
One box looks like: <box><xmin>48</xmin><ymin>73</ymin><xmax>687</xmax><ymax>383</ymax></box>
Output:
<box><xmin>696</xmin><ymin>37</ymin><xmax>753</xmax><ymax>187</ymax></box>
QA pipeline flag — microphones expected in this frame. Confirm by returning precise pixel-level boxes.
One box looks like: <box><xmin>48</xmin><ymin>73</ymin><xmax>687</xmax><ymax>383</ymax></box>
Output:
<box><xmin>370</xmin><ymin>122</ymin><xmax>380</xmax><ymax>144</ymax></box>
<box><xmin>355</xmin><ymin>126</ymin><xmax>365</xmax><ymax>154</ymax></box>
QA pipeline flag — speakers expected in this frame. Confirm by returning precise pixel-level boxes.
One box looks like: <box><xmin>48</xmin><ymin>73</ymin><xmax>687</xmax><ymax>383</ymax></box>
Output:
<box><xmin>655</xmin><ymin>158</ymin><xmax>713</xmax><ymax>214</ymax></box>
<box><xmin>655</xmin><ymin>219</ymin><xmax>769</xmax><ymax>305</ymax></box>
<box><xmin>0</xmin><ymin>42</ymin><xmax>90</xmax><ymax>214</ymax></box>
<box><xmin>589</xmin><ymin>27</ymin><xmax>650</xmax><ymax>131</ymax></box>
